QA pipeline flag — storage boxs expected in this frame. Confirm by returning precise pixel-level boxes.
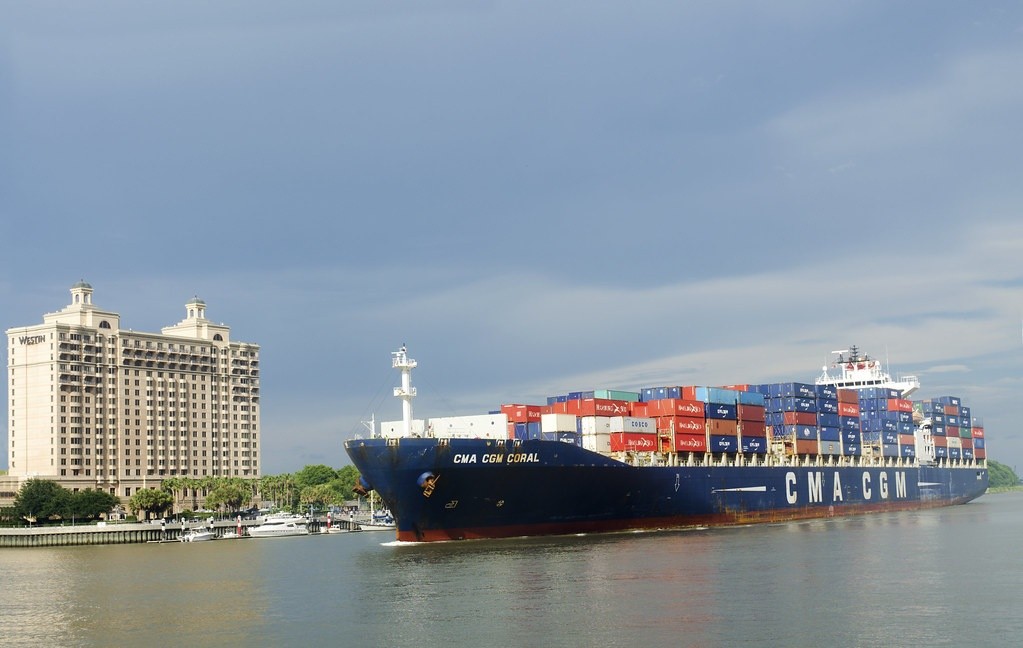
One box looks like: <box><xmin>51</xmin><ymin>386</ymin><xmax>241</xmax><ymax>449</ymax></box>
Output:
<box><xmin>487</xmin><ymin>380</ymin><xmax>987</xmax><ymax>468</ymax></box>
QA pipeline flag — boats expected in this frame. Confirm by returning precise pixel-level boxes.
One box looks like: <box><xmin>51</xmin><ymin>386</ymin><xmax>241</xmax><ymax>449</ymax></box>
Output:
<box><xmin>177</xmin><ymin>527</ymin><xmax>217</xmax><ymax>542</ymax></box>
<box><xmin>246</xmin><ymin>520</ymin><xmax>310</xmax><ymax>538</ymax></box>
<box><xmin>319</xmin><ymin>525</ymin><xmax>349</xmax><ymax>534</ymax></box>
<box><xmin>359</xmin><ymin>510</ymin><xmax>396</xmax><ymax>530</ymax></box>
<box><xmin>344</xmin><ymin>342</ymin><xmax>988</xmax><ymax>545</ymax></box>
